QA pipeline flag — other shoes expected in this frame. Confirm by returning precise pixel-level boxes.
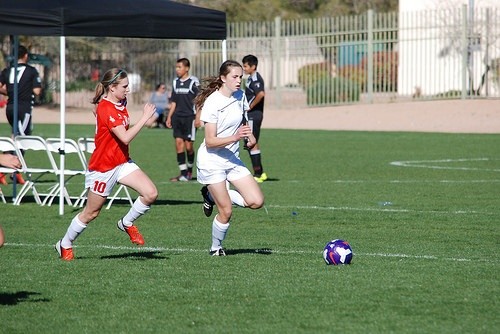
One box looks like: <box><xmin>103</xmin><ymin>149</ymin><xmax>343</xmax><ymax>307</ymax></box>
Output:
<box><xmin>10</xmin><ymin>172</ymin><xmax>25</xmax><ymax>184</ymax></box>
<box><xmin>187</xmin><ymin>171</ymin><xmax>192</xmax><ymax>179</ymax></box>
<box><xmin>169</xmin><ymin>174</ymin><xmax>188</xmax><ymax>182</ymax></box>
<box><xmin>260</xmin><ymin>172</ymin><xmax>268</xmax><ymax>180</ymax></box>
<box><xmin>0</xmin><ymin>172</ymin><xmax>8</xmax><ymax>185</ymax></box>
<box><xmin>254</xmin><ymin>176</ymin><xmax>263</xmax><ymax>182</ymax></box>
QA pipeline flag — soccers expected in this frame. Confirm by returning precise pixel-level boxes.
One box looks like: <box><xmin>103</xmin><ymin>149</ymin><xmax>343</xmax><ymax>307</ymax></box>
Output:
<box><xmin>322</xmin><ymin>239</ymin><xmax>353</xmax><ymax>265</ymax></box>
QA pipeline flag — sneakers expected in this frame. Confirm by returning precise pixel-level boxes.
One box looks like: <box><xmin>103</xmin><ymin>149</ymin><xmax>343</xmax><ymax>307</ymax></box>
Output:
<box><xmin>200</xmin><ymin>185</ymin><xmax>215</xmax><ymax>216</ymax></box>
<box><xmin>55</xmin><ymin>239</ymin><xmax>75</xmax><ymax>259</ymax></box>
<box><xmin>117</xmin><ymin>217</ymin><xmax>145</xmax><ymax>245</ymax></box>
<box><xmin>209</xmin><ymin>246</ymin><xmax>226</xmax><ymax>256</ymax></box>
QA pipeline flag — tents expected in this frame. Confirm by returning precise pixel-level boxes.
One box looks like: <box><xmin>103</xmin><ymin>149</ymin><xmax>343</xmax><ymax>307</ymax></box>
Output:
<box><xmin>0</xmin><ymin>0</ymin><xmax>227</xmax><ymax>216</ymax></box>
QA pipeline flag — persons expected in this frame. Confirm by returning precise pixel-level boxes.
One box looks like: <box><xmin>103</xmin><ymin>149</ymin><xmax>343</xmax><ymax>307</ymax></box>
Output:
<box><xmin>193</xmin><ymin>60</ymin><xmax>264</xmax><ymax>256</ymax></box>
<box><xmin>0</xmin><ymin>43</ymin><xmax>265</xmax><ymax>250</ymax></box>
<box><xmin>54</xmin><ymin>68</ymin><xmax>158</xmax><ymax>260</ymax></box>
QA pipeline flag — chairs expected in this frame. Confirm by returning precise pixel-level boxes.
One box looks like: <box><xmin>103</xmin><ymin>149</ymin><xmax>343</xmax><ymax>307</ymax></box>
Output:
<box><xmin>0</xmin><ymin>135</ymin><xmax>133</xmax><ymax>210</ymax></box>
